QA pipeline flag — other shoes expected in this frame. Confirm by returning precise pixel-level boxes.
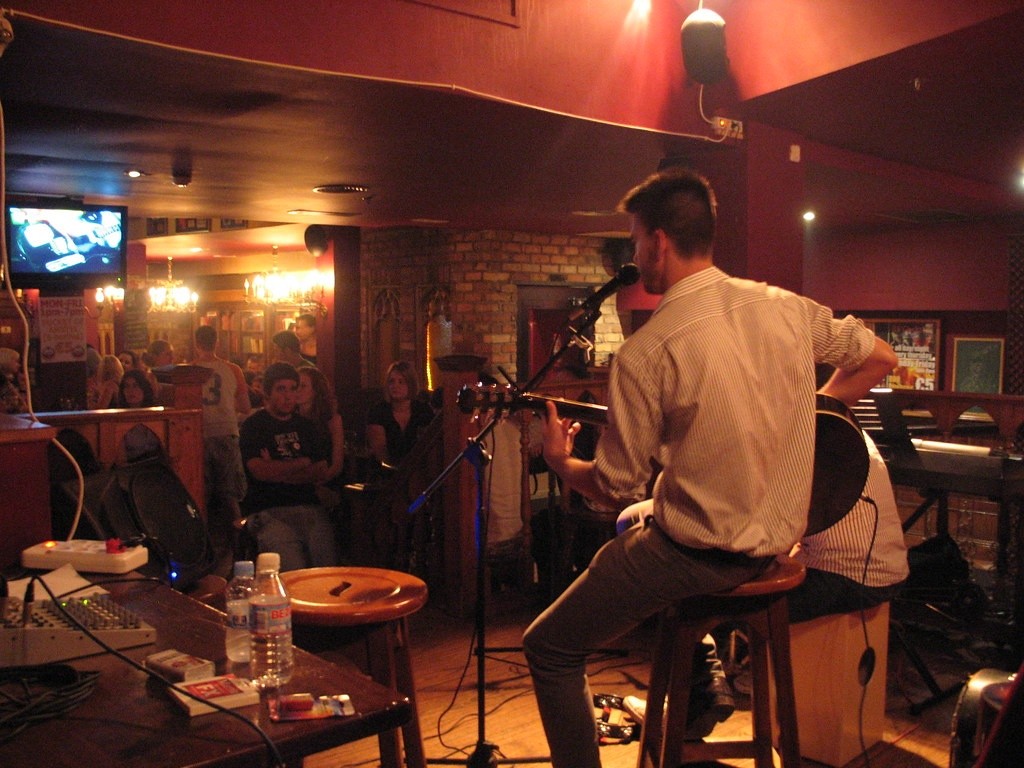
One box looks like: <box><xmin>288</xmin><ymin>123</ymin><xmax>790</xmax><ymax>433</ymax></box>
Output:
<box><xmin>683</xmin><ymin>678</ymin><xmax>734</xmax><ymax>742</ymax></box>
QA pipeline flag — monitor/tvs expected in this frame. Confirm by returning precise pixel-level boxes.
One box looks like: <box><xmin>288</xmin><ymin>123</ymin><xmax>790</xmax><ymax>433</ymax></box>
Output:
<box><xmin>5</xmin><ymin>201</ymin><xmax>128</xmax><ymax>284</ymax></box>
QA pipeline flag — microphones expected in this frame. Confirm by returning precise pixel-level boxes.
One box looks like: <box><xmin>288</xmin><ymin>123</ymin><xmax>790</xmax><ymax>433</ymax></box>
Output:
<box><xmin>567</xmin><ymin>263</ymin><xmax>641</xmax><ymax>321</ymax></box>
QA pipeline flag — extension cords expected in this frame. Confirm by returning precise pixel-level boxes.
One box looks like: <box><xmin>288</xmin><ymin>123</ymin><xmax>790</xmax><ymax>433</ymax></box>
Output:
<box><xmin>21</xmin><ymin>539</ymin><xmax>148</xmax><ymax>574</ymax></box>
<box><xmin>710</xmin><ymin>116</ymin><xmax>742</xmax><ymax>132</ymax></box>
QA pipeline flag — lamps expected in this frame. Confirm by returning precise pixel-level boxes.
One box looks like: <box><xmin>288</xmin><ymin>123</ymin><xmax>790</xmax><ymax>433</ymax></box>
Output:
<box><xmin>80</xmin><ymin>283</ymin><xmax>125</xmax><ymax>320</ymax></box>
<box><xmin>243</xmin><ymin>249</ymin><xmax>323</xmax><ymax>309</ymax></box>
<box><xmin>146</xmin><ymin>255</ymin><xmax>198</xmax><ymax>314</ymax></box>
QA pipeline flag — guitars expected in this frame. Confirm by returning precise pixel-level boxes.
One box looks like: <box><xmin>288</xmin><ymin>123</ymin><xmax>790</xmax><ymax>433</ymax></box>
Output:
<box><xmin>456</xmin><ymin>381</ymin><xmax>870</xmax><ymax>539</ymax></box>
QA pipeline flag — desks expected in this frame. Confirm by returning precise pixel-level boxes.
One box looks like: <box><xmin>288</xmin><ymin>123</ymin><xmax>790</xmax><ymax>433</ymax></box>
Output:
<box><xmin>1</xmin><ymin>565</ymin><xmax>412</xmax><ymax>768</ymax></box>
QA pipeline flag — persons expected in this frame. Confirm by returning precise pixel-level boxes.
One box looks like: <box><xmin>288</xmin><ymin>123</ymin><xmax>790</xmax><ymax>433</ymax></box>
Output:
<box><xmin>523</xmin><ymin>163</ymin><xmax>897</xmax><ymax>768</ymax></box>
<box><xmin>0</xmin><ymin>313</ymin><xmax>436</xmax><ymax>574</ymax></box>
<box><xmin>712</xmin><ymin>414</ymin><xmax>910</xmax><ymax>702</ymax></box>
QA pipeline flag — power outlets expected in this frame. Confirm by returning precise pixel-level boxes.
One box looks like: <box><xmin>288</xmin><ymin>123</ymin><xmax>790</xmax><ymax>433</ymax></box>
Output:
<box><xmin>712</xmin><ymin>117</ymin><xmax>744</xmax><ymax>140</ymax></box>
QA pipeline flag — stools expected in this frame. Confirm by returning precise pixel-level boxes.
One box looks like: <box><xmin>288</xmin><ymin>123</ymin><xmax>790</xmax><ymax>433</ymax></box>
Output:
<box><xmin>635</xmin><ymin>555</ymin><xmax>806</xmax><ymax>768</ymax></box>
<box><xmin>274</xmin><ymin>567</ymin><xmax>426</xmax><ymax>768</ymax></box>
<box><xmin>763</xmin><ymin>599</ymin><xmax>889</xmax><ymax>768</ymax></box>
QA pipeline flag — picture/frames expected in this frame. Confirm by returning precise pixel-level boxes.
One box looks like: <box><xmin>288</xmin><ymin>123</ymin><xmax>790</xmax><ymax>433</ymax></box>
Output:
<box><xmin>951</xmin><ymin>337</ymin><xmax>1006</xmax><ymax>394</ymax></box>
<box><xmin>855</xmin><ymin>319</ymin><xmax>943</xmax><ymax>393</ymax></box>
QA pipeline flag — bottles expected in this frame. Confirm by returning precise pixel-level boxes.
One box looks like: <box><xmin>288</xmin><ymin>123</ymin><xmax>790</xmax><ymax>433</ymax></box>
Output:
<box><xmin>248</xmin><ymin>552</ymin><xmax>295</xmax><ymax>690</ymax></box>
<box><xmin>224</xmin><ymin>560</ymin><xmax>264</xmax><ymax>661</ymax></box>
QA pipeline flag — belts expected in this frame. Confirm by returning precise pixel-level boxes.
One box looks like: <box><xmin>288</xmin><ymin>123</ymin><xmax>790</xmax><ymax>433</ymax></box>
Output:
<box><xmin>677</xmin><ymin>535</ymin><xmax>773</xmax><ymax>567</ymax></box>
